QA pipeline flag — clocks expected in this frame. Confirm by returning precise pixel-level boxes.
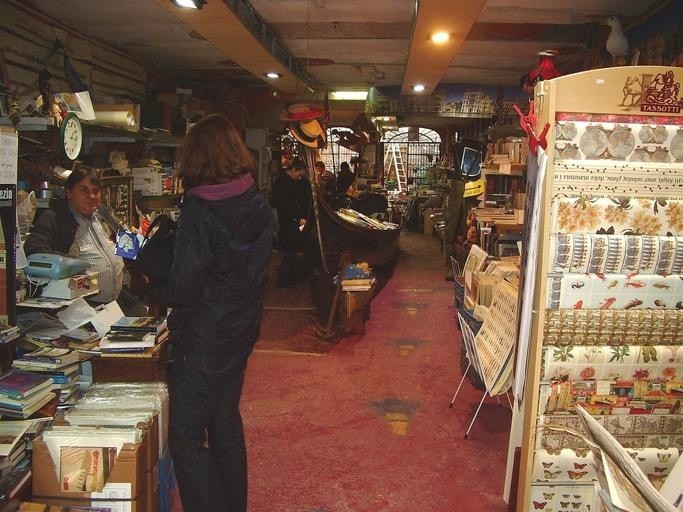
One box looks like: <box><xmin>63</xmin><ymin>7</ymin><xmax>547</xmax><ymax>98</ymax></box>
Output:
<box><xmin>59</xmin><ymin>113</ymin><xmax>84</xmax><ymax>163</ymax></box>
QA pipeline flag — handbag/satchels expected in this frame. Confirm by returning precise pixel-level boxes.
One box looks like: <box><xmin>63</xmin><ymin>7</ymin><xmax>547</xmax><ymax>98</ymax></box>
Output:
<box><xmin>135</xmin><ymin>214</ymin><xmax>176</xmax><ymax>281</ymax></box>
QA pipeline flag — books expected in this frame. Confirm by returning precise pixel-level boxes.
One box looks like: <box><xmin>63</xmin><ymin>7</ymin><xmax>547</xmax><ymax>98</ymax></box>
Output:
<box><xmin>0</xmin><ymin>302</ymin><xmax>167</xmax><ymax>501</ymax></box>
<box><xmin>480</xmin><ymin>138</ymin><xmax>526</xmax><ymax>175</ymax></box>
<box><xmin>564</xmin><ymin>403</ymin><xmax>683</xmax><ymax>512</ymax></box>
<box><xmin>338</xmin><ymin>208</ymin><xmax>398</xmax><ymax>233</ymax></box>
<box><xmin>132</xmin><ymin>158</ymin><xmax>182</xmax><ymax>198</ymax></box>
<box><xmin>459</xmin><ymin>243</ymin><xmax>530</xmax><ymax>397</ymax></box>
<box><xmin>472</xmin><ymin>192</ymin><xmax>526</xmax><ymax>242</ymax></box>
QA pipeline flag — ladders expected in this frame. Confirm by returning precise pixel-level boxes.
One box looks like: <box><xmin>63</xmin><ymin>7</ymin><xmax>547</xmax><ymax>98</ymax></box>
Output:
<box><xmin>384</xmin><ymin>143</ymin><xmax>407</xmax><ymax>192</ymax></box>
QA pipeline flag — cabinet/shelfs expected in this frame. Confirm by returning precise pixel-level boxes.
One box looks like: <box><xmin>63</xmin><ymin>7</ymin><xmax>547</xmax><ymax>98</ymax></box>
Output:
<box><xmin>472</xmin><ymin>161</ymin><xmax>525</xmax><ymax>256</ymax></box>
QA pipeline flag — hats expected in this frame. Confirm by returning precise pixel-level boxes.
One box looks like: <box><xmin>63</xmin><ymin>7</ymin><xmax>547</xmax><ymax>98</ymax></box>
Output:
<box><xmin>279</xmin><ymin>102</ymin><xmax>326</xmax><ymax>149</ymax></box>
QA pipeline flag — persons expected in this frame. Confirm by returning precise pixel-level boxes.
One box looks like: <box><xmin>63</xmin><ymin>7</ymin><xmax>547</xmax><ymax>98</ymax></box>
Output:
<box><xmin>22</xmin><ymin>165</ymin><xmax>124</xmax><ymax>302</ymax></box>
<box><xmin>315</xmin><ymin>161</ymin><xmax>337</xmax><ymax>199</ymax></box>
<box><xmin>271</xmin><ymin>161</ymin><xmax>310</xmax><ymax>276</ymax></box>
<box><xmin>337</xmin><ymin>162</ymin><xmax>354</xmax><ymax>191</ymax></box>
<box><xmin>142</xmin><ymin>113</ymin><xmax>277</xmax><ymax>512</ymax></box>
